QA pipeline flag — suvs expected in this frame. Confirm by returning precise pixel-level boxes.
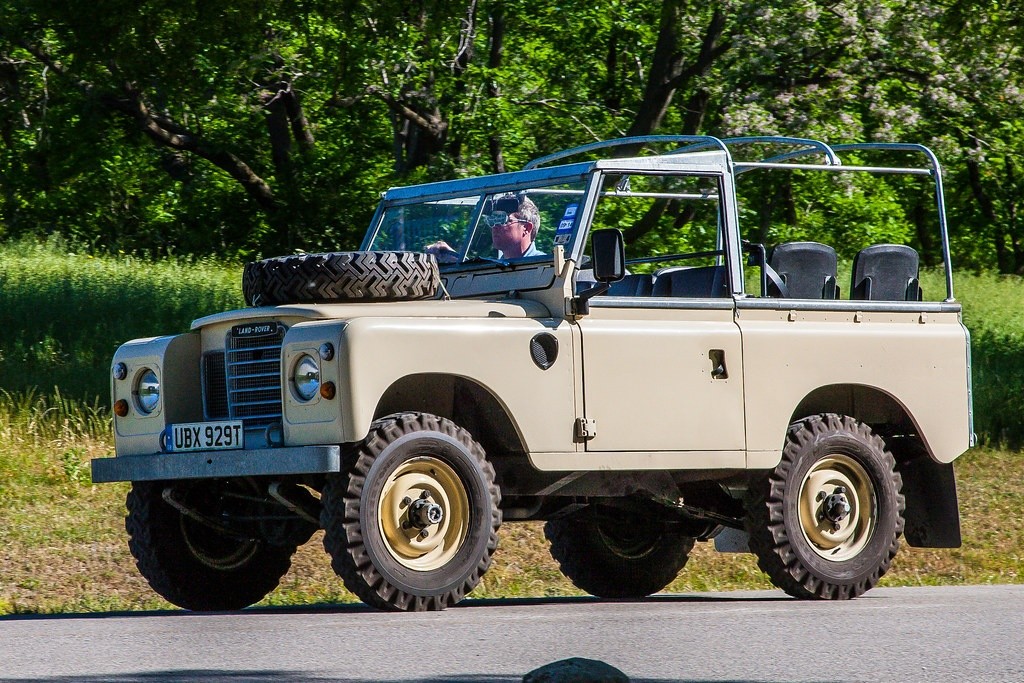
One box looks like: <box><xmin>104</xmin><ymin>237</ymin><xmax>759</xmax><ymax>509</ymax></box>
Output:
<box><xmin>92</xmin><ymin>134</ymin><xmax>978</xmax><ymax>613</ymax></box>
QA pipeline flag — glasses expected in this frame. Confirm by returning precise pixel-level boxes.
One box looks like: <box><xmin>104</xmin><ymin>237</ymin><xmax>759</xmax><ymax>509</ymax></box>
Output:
<box><xmin>483</xmin><ymin>211</ymin><xmax>529</xmax><ymax>228</ymax></box>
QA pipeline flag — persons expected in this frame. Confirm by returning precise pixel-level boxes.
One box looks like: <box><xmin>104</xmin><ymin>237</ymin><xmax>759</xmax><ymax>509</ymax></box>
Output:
<box><xmin>424</xmin><ymin>194</ymin><xmax>548</xmax><ymax>263</ymax></box>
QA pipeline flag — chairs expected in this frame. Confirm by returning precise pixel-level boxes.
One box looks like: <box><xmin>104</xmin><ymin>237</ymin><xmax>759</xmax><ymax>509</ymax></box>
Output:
<box><xmin>591</xmin><ymin>273</ymin><xmax>657</xmax><ymax>297</ymax></box>
<box><xmin>850</xmin><ymin>243</ymin><xmax>923</xmax><ymax>301</ymax></box>
<box><xmin>766</xmin><ymin>242</ymin><xmax>841</xmax><ymax>300</ymax></box>
<box><xmin>651</xmin><ymin>266</ymin><xmax>730</xmax><ymax>298</ymax></box>
